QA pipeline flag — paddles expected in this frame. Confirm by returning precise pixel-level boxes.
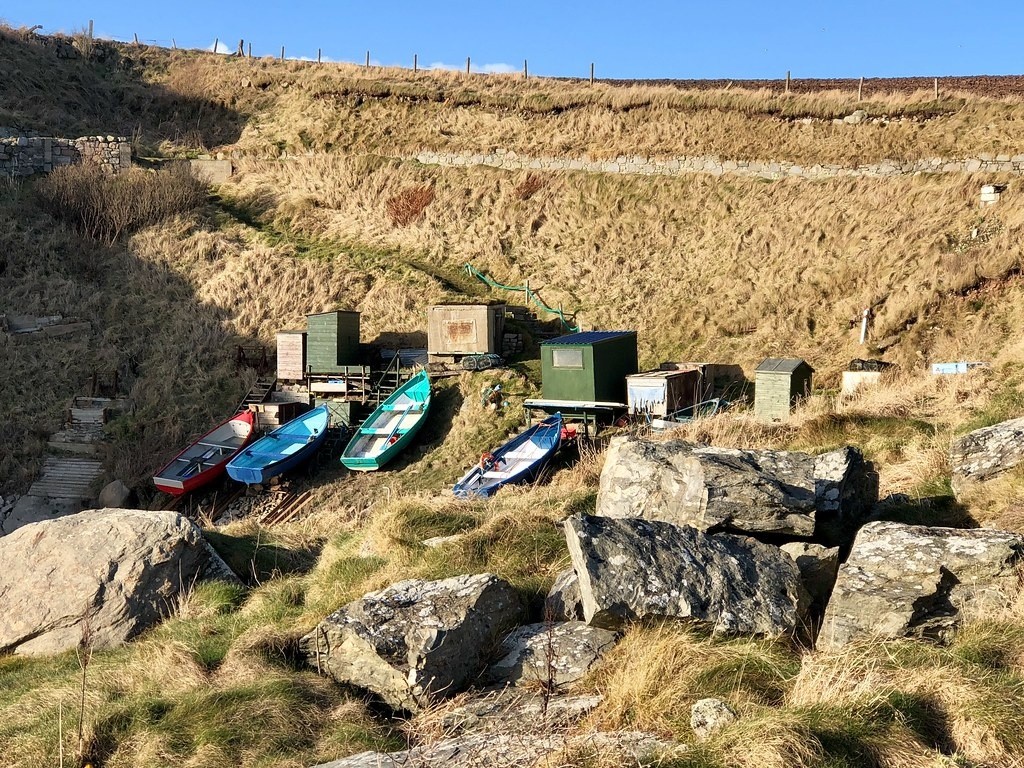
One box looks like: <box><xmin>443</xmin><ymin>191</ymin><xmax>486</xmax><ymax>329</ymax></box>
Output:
<box><xmin>373</xmin><ymin>405</ymin><xmax>413</xmax><ymax>457</ymax></box>
<box><xmin>180</xmin><ymin>446</ymin><xmax>220</xmax><ymax>478</ymax></box>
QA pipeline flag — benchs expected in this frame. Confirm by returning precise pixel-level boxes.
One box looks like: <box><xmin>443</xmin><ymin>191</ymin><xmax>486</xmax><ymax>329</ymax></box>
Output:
<box><xmin>178</xmin><ymin>456</ymin><xmax>219</xmax><ymax>465</ymax></box>
<box><xmin>274</xmin><ymin>433</ymin><xmax>312</xmax><ymax>439</ymax></box>
<box><xmin>381</xmin><ymin>404</ymin><xmax>424</xmax><ymax>411</ymax></box>
<box><xmin>483</xmin><ymin>471</ymin><xmax>514</xmax><ymax>478</ymax></box>
<box><xmin>360</xmin><ymin>428</ymin><xmax>409</xmax><ymax>434</ymax></box>
<box><xmin>504</xmin><ymin>452</ymin><xmax>543</xmax><ymax>460</ymax></box>
<box><xmin>246</xmin><ymin>451</ymin><xmax>289</xmax><ymax>459</ymax></box>
<box><xmin>198</xmin><ymin>439</ymin><xmax>240</xmax><ymax>449</ymax></box>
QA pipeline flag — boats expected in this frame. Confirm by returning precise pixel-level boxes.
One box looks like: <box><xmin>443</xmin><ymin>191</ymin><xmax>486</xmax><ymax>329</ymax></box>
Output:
<box><xmin>339</xmin><ymin>369</ymin><xmax>433</xmax><ymax>473</ymax></box>
<box><xmin>151</xmin><ymin>407</ymin><xmax>254</xmax><ymax>499</ymax></box>
<box><xmin>445</xmin><ymin>410</ymin><xmax>563</xmax><ymax>501</ymax></box>
<box><xmin>609</xmin><ymin>394</ymin><xmax>720</xmax><ymax>445</ymax></box>
<box><xmin>224</xmin><ymin>402</ymin><xmax>331</xmax><ymax>491</ymax></box>
